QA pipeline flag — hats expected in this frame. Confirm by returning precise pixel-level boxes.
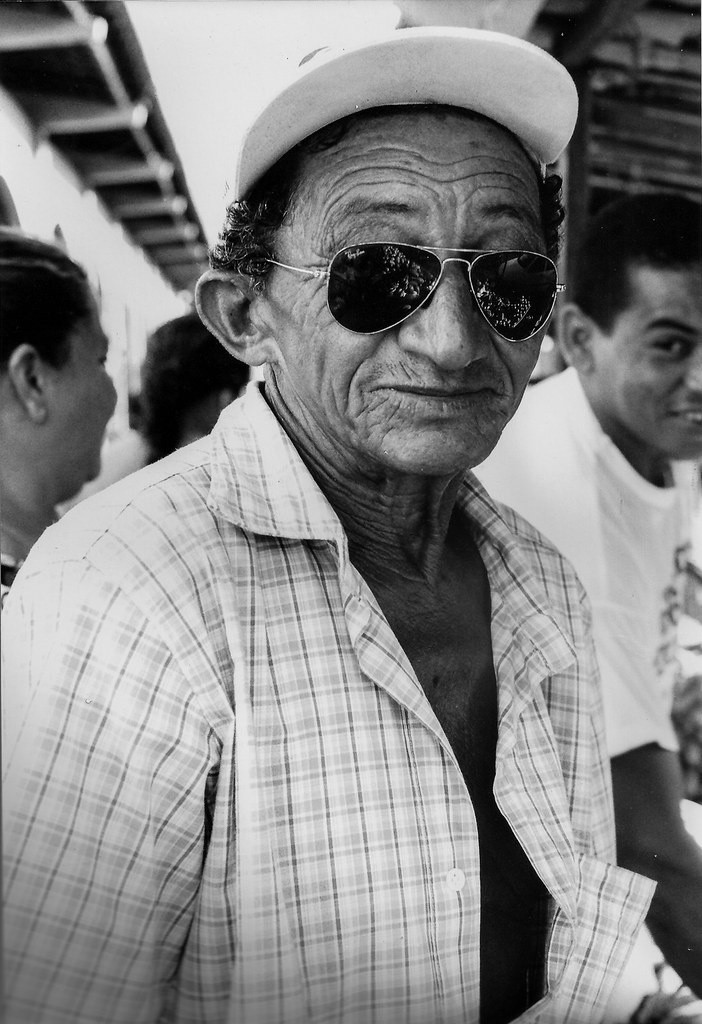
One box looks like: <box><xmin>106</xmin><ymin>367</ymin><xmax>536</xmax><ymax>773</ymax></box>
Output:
<box><xmin>234</xmin><ymin>25</ymin><xmax>582</xmax><ymax>203</ymax></box>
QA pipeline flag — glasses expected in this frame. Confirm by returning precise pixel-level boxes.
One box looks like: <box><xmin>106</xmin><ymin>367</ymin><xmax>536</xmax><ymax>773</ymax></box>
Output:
<box><xmin>249</xmin><ymin>242</ymin><xmax>568</xmax><ymax>344</ymax></box>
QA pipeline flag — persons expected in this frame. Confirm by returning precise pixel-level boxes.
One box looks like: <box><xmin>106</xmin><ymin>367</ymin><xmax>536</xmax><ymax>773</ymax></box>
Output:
<box><xmin>465</xmin><ymin>192</ymin><xmax>702</xmax><ymax>995</ymax></box>
<box><xmin>0</xmin><ymin>230</ymin><xmax>118</xmax><ymax>600</ymax></box>
<box><xmin>0</xmin><ymin>23</ymin><xmax>702</xmax><ymax>1024</ymax></box>
<box><xmin>127</xmin><ymin>313</ymin><xmax>250</xmax><ymax>465</ymax></box>
